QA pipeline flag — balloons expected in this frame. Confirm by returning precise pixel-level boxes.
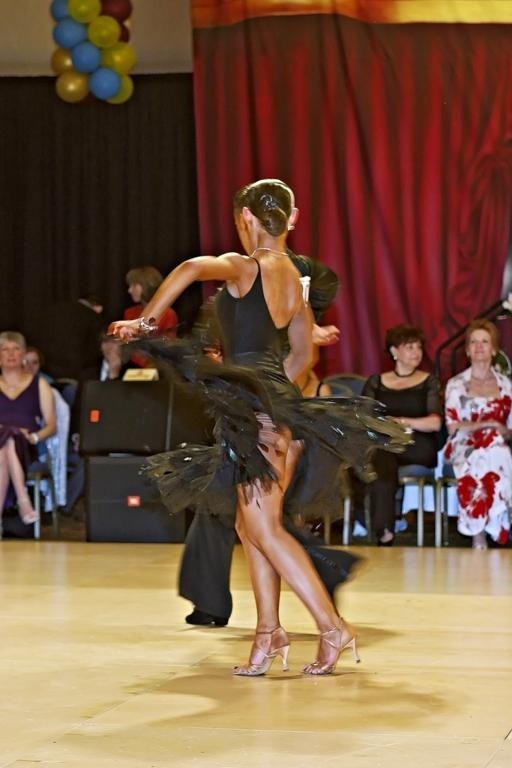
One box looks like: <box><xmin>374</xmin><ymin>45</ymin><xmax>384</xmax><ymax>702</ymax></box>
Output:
<box><xmin>49</xmin><ymin>0</ymin><xmax>136</xmax><ymax>106</ymax></box>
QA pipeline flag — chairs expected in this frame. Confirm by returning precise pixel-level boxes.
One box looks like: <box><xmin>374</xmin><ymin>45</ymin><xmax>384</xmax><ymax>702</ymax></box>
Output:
<box><xmin>24</xmin><ymin>432</ymin><xmax>61</xmax><ymax>540</ymax></box>
<box><xmin>363</xmin><ymin>462</ymin><xmax>437</xmax><ymax>548</ymax></box>
<box><xmin>434</xmin><ymin>464</ymin><xmax>459</xmax><ymax>550</ymax></box>
<box><xmin>323</xmin><ymin>382</ymin><xmax>358</xmax><ymax>545</ymax></box>
<box><xmin>322</xmin><ymin>372</ymin><xmax>368</xmax><ymax>394</ymax></box>
<box><xmin>56</xmin><ymin>375</ymin><xmax>79</xmax><ymax>405</ymax></box>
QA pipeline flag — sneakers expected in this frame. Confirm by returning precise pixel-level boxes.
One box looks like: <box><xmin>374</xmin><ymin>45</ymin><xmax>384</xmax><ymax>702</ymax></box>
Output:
<box><xmin>352</xmin><ymin>520</ymin><xmax>368</xmax><ymax>537</ymax></box>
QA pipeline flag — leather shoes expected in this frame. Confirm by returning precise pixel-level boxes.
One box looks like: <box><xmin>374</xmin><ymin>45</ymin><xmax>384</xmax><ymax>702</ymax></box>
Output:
<box><xmin>185</xmin><ymin>606</ymin><xmax>228</xmax><ymax>626</ymax></box>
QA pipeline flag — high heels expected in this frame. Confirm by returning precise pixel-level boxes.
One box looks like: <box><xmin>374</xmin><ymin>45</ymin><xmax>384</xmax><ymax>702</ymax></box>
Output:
<box><xmin>233</xmin><ymin>626</ymin><xmax>290</xmax><ymax>676</ymax></box>
<box><xmin>302</xmin><ymin>617</ymin><xmax>360</xmax><ymax>675</ymax></box>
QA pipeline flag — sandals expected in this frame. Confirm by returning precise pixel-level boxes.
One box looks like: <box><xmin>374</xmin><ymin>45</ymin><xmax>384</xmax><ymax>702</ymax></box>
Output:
<box><xmin>473</xmin><ymin>534</ymin><xmax>488</xmax><ymax>552</ymax></box>
<box><xmin>18</xmin><ymin>499</ymin><xmax>40</xmax><ymax>524</ymax></box>
<box><xmin>395</xmin><ymin>518</ymin><xmax>408</xmax><ymax>534</ymax></box>
<box><xmin>378</xmin><ymin>532</ymin><xmax>394</xmax><ymax>546</ymax></box>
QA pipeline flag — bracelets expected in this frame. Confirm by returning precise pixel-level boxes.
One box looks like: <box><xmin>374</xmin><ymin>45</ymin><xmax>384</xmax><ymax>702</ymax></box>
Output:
<box><xmin>138</xmin><ymin>316</ymin><xmax>160</xmax><ymax>334</ymax></box>
<box><xmin>399</xmin><ymin>416</ymin><xmax>404</xmax><ymax>425</ymax></box>
<box><xmin>31</xmin><ymin>431</ymin><xmax>40</xmax><ymax>446</ymax></box>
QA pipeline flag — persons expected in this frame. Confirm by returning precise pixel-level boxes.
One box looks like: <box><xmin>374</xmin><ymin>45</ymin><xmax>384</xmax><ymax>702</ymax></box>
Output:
<box><xmin>56</xmin><ymin>284</ymin><xmax>111</xmax><ymax>382</ymax></box>
<box><xmin>176</xmin><ymin>182</ymin><xmax>356</xmax><ymax>626</ymax></box>
<box><xmin>442</xmin><ymin>318</ymin><xmax>511</xmax><ymax>549</ymax></box>
<box><xmin>294</xmin><ymin>368</ymin><xmax>333</xmax><ymax>402</ymax></box>
<box><xmin>105</xmin><ymin>177</ymin><xmax>419</xmax><ymax>677</ymax></box>
<box><xmin>23</xmin><ymin>345</ymin><xmax>91</xmax><ymax>528</ymax></box>
<box><xmin>68</xmin><ymin>330</ymin><xmax>148</xmax><ymax>457</ymax></box>
<box><xmin>0</xmin><ymin>331</ymin><xmax>58</xmax><ymax>536</ymax></box>
<box><xmin>109</xmin><ymin>263</ymin><xmax>178</xmax><ymax>367</ymax></box>
<box><xmin>350</xmin><ymin>321</ymin><xmax>445</xmax><ymax>546</ymax></box>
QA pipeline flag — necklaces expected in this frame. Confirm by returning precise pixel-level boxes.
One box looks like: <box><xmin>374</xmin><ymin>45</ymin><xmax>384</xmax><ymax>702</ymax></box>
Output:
<box><xmin>249</xmin><ymin>246</ymin><xmax>290</xmax><ymax>257</ymax></box>
<box><xmin>393</xmin><ymin>369</ymin><xmax>415</xmax><ymax>378</ymax></box>
